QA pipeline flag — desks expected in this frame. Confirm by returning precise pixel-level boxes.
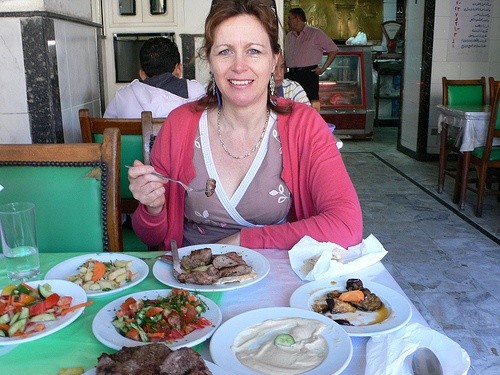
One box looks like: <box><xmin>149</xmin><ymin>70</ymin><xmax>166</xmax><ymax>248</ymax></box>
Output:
<box><xmin>435</xmin><ymin>103</ymin><xmax>500</xmax><ymax>210</ymax></box>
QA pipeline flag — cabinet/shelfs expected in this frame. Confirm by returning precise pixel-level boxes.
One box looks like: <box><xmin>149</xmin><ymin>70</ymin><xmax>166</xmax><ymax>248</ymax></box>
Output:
<box><xmin>372</xmin><ymin>48</ymin><xmax>403</xmax><ymax>128</ymax></box>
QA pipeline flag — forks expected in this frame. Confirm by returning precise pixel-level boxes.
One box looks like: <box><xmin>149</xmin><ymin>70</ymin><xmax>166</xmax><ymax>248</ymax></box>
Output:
<box><xmin>124</xmin><ymin>164</ymin><xmax>212</xmax><ymax>193</ymax></box>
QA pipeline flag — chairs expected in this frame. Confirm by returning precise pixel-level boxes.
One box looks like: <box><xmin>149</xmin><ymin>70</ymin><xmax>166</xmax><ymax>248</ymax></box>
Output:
<box><xmin>441</xmin><ymin>77</ymin><xmax>500</xmax><ymax>217</ymax></box>
<box><xmin>0</xmin><ymin>108</ymin><xmax>167</xmax><ymax>252</ymax></box>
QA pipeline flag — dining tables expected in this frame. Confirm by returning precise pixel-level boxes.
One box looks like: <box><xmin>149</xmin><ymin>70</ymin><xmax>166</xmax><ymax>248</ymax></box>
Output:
<box><xmin>0</xmin><ymin>251</ymin><xmax>428</xmax><ymax>375</ymax></box>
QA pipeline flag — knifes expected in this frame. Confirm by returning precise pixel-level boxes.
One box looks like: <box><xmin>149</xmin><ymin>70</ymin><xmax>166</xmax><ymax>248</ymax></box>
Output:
<box><xmin>171</xmin><ymin>239</ymin><xmax>183</xmax><ymax>277</ymax></box>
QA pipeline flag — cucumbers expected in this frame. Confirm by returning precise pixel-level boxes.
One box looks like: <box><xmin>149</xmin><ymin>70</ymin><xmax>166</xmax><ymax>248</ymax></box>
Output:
<box><xmin>275</xmin><ymin>334</ymin><xmax>296</xmax><ymax>346</ymax></box>
<box><xmin>0</xmin><ymin>307</ymin><xmax>55</xmax><ymax>337</ymax></box>
<box><xmin>38</xmin><ymin>284</ymin><xmax>52</xmax><ymax>299</ymax></box>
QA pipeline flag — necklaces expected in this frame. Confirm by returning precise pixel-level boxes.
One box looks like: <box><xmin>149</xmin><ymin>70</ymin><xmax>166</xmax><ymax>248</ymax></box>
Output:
<box><xmin>217</xmin><ymin>102</ymin><xmax>271</xmax><ymax>160</ymax></box>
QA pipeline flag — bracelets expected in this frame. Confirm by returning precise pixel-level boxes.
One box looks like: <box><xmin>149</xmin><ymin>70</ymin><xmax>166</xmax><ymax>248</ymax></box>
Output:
<box><xmin>322</xmin><ymin>68</ymin><xmax>325</xmax><ymax>72</ymax></box>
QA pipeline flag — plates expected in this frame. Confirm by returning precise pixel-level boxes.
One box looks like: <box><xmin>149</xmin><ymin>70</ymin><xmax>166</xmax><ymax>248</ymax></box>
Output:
<box><xmin>91</xmin><ymin>289</ymin><xmax>223</xmax><ymax>351</ymax></box>
<box><xmin>79</xmin><ymin>360</ymin><xmax>224</xmax><ymax>375</ymax></box>
<box><xmin>152</xmin><ymin>244</ymin><xmax>277</xmax><ymax>293</ymax></box>
<box><xmin>290</xmin><ymin>278</ymin><xmax>412</xmax><ymax>337</ymax></box>
<box><xmin>44</xmin><ymin>252</ymin><xmax>149</xmax><ymax>297</ymax></box>
<box><xmin>0</xmin><ymin>278</ymin><xmax>88</xmax><ymax>345</ymax></box>
<box><xmin>209</xmin><ymin>306</ymin><xmax>354</xmax><ymax>375</ymax></box>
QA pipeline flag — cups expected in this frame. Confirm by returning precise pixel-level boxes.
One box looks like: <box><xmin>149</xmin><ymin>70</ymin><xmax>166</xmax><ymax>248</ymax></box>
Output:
<box><xmin>0</xmin><ymin>202</ymin><xmax>41</xmax><ymax>284</ymax></box>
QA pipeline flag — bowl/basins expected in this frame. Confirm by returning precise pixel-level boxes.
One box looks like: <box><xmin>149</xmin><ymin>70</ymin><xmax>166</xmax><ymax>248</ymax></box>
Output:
<box><xmin>327</xmin><ymin>123</ymin><xmax>336</xmax><ymax>133</ymax></box>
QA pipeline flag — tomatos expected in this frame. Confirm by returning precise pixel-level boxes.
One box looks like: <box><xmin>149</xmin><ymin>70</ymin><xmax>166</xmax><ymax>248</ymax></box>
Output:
<box><xmin>0</xmin><ymin>285</ymin><xmax>60</xmax><ymax>316</ymax></box>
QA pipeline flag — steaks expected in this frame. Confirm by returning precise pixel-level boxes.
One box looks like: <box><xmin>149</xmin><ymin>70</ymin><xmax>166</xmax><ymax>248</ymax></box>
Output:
<box><xmin>205</xmin><ymin>178</ymin><xmax>216</xmax><ymax>197</ymax></box>
<box><xmin>178</xmin><ymin>248</ymin><xmax>253</xmax><ymax>285</ymax></box>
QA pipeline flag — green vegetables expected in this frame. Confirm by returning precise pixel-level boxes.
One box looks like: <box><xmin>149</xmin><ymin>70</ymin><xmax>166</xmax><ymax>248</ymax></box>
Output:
<box><xmin>124</xmin><ymin>289</ymin><xmax>210</xmax><ymax>344</ymax></box>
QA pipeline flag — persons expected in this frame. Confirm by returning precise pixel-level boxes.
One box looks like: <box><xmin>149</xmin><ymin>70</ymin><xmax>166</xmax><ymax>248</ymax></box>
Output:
<box><xmin>102</xmin><ymin>37</ymin><xmax>207</xmax><ymax>120</ymax></box>
<box><xmin>283</xmin><ymin>8</ymin><xmax>339</xmax><ymax>108</ymax></box>
<box><xmin>271</xmin><ymin>49</ymin><xmax>311</xmax><ymax>106</ymax></box>
<box><xmin>127</xmin><ymin>0</ymin><xmax>363</xmax><ymax>255</ymax></box>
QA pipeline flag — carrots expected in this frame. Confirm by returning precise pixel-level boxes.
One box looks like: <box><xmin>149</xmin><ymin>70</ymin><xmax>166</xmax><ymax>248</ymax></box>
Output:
<box><xmin>93</xmin><ymin>263</ymin><xmax>105</xmax><ymax>280</ymax></box>
<box><xmin>338</xmin><ymin>290</ymin><xmax>364</xmax><ymax>301</ymax></box>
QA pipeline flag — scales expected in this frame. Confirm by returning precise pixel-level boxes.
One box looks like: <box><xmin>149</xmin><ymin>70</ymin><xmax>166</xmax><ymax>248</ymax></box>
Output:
<box><xmin>372</xmin><ymin>21</ymin><xmax>403</xmax><ymax>59</ymax></box>
